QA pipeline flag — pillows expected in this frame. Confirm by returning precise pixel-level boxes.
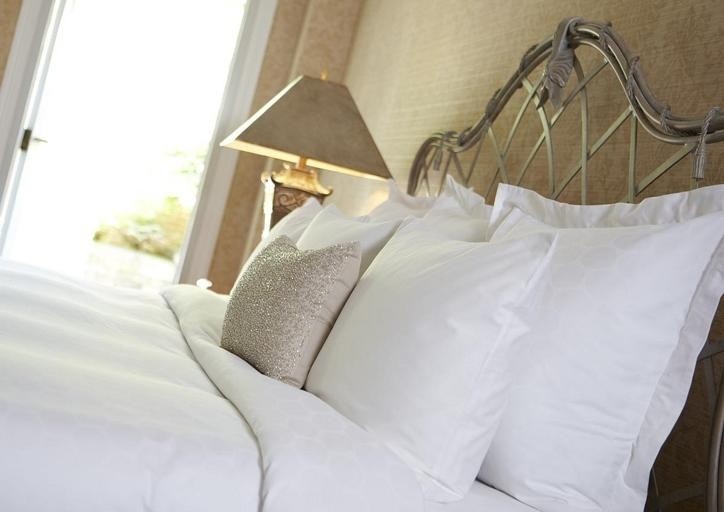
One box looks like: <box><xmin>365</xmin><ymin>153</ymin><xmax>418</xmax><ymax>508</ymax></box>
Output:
<box><xmin>296</xmin><ymin>203</ymin><xmax>402</xmax><ymax>277</ymax></box>
<box><xmin>476</xmin><ymin>182</ymin><xmax>724</xmax><ymax>512</ymax></box>
<box><xmin>359</xmin><ymin>181</ymin><xmax>436</xmax><ymax>221</ymax></box>
<box><xmin>219</xmin><ymin>234</ymin><xmax>362</xmax><ymax>388</ymax></box>
<box><xmin>219</xmin><ymin>195</ymin><xmax>322</xmax><ymax>297</ymax></box>
<box><xmin>424</xmin><ymin>176</ymin><xmax>493</xmax><ymax>219</ymax></box>
<box><xmin>304</xmin><ymin>214</ymin><xmax>560</xmax><ymax>495</ymax></box>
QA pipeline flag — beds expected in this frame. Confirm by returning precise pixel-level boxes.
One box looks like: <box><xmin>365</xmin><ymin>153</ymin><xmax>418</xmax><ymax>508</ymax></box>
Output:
<box><xmin>2</xmin><ymin>20</ymin><xmax>723</xmax><ymax>512</ymax></box>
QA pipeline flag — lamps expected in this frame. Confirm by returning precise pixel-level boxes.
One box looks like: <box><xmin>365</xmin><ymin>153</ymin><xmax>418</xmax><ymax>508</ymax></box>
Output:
<box><xmin>219</xmin><ymin>74</ymin><xmax>392</xmax><ymax>242</ymax></box>
